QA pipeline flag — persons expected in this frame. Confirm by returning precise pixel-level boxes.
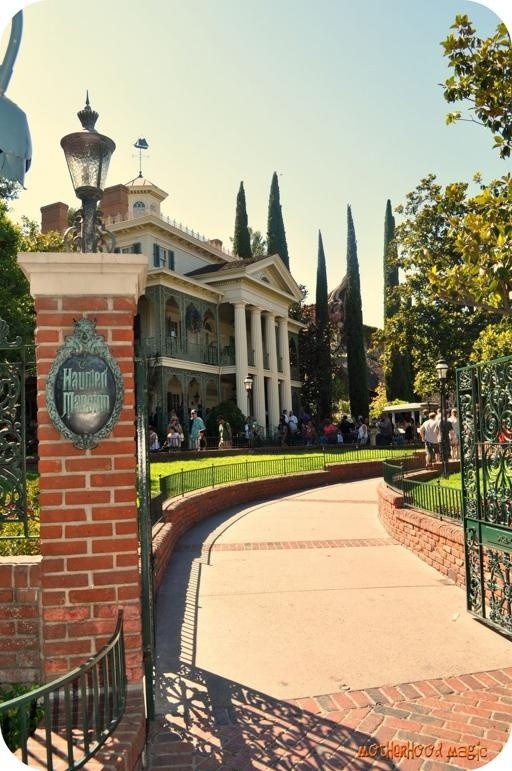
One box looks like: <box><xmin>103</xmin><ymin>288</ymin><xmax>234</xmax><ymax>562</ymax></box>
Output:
<box><xmin>188</xmin><ymin>407</ymin><xmax>207</xmax><ymax>450</ymax></box>
<box><xmin>148</xmin><ymin>424</ymin><xmax>159</xmax><ymax>451</ymax></box>
<box><xmin>243</xmin><ymin>420</ymin><xmax>257</xmax><ymax>445</ymax></box>
<box><xmin>277</xmin><ymin>406</ymin><xmax>512</xmax><ymax>475</ymax></box>
<box><xmin>252</xmin><ymin>421</ymin><xmax>265</xmax><ymax>440</ymax></box>
<box><xmin>163</xmin><ymin>423</ymin><xmax>182</xmax><ymax>451</ymax></box>
<box><xmin>215</xmin><ymin>414</ymin><xmax>233</xmax><ymax>449</ymax></box>
<box><xmin>168</xmin><ymin>413</ymin><xmax>184</xmax><ymax>446</ymax></box>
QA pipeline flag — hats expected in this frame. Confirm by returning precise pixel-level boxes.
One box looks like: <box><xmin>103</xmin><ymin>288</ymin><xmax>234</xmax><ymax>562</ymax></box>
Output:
<box><xmin>217</xmin><ymin>415</ymin><xmax>224</xmax><ymax>421</ymax></box>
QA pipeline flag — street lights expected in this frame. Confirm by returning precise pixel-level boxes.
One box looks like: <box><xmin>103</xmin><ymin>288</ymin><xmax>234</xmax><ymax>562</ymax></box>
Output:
<box><xmin>244</xmin><ymin>375</ymin><xmax>253</xmax><ymax>418</ymax></box>
<box><xmin>432</xmin><ymin>355</ymin><xmax>452</xmax><ymax>477</ymax></box>
